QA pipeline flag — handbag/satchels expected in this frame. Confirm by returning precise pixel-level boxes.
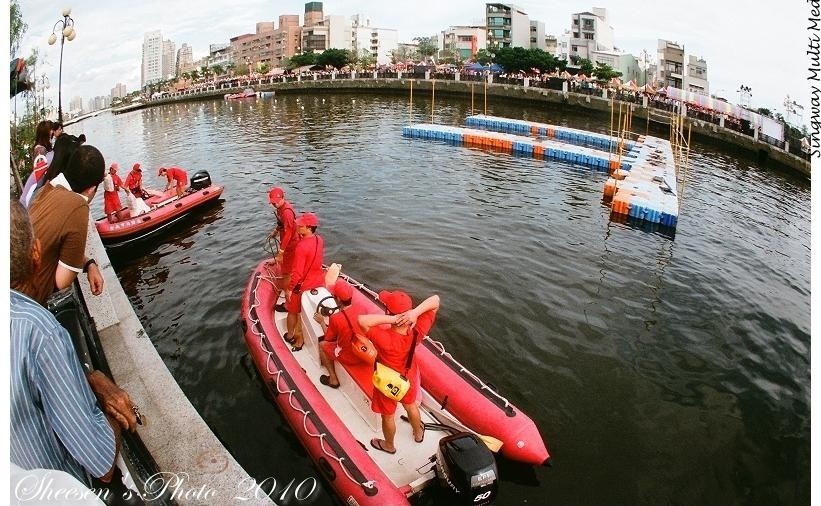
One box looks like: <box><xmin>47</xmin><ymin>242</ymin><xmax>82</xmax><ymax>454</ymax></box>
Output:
<box><xmin>350</xmin><ymin>333</ymin><xmax>378</xmax><ymax>363</ymax></box>
<box><xmin>372</xmin><ymin>362</ymin><xmax>411</xmax><ymax>400</ymax></box>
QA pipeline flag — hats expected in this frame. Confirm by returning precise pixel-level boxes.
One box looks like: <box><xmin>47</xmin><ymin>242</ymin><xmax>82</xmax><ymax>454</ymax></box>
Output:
<box><xmin>111</xmin><ymin>163</ymin><xmax>167</xmax><ymax>176</ymax></box>
<box><xmin>269</xmin><ymin>188</ymin><xmax>412</xmax><ymax>315</ymax></box>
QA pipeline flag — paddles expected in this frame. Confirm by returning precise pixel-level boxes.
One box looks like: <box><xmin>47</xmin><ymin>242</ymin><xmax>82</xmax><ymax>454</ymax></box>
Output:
<box><xmin>422</xmin><ymin>401</ymin><xmax>504</xmax><ymax>453</ymax></box>
<box><xmin>324</xmin><ymin>263</ymin><xmax>343</xmax><ymax>287</ymax></box>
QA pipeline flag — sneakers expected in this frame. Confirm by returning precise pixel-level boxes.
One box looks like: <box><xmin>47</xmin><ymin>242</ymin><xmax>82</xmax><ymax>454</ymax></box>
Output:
<box><xmin>284</xmin><ymin>333</ymin><xmax>296</xmax><ymax>344</ymax></box>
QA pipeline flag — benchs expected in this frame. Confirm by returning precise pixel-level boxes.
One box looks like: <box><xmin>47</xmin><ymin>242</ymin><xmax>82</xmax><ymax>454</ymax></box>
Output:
<box><xmin>151</xmin><ymin>191</ymin><xmax>184</xmax><ymax>210</ymax></box>
<box><xmin>334</xmin><ymin>351</ymin><xmax>401</xmax><ymax>432</ymax></box>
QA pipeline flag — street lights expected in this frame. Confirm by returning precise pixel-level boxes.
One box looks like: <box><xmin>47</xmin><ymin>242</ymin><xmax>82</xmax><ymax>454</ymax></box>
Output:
<box><xmin>484</xmin><ymin>28</ymin><xmax>498</xmax><ymax>83</ymax></box>
<box><xmin>46</xmin><ymin>6</ymin><xmax>75</xmax><ymax>129</ymax></box>
<box><xmin>781</xmin><ymin>92</ymin><xmax>796</xmax><ymax>152</ymax></box>
<box><xmin>638</xmin><ymin>47</ymin><xmax>652</xmax><ymax>107</ymax></box>
<box><xmin>736</xmin><ymin>84</ymin><xmax>752</xmax><ymax>108</ymax></box>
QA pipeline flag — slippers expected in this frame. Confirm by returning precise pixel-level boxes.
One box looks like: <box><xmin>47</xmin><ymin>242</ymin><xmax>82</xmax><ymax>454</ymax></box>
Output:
<box><xmin>320</xmin><ymin>374</ymin><xmax>340</xmax><ymax>388</ymax></box>
<box><xmin>371</xmin><ymin>439</ymin><xmax>396</xmax><ymax>454</ymax></box>
<box><xmin>415</xmin><ymin>421</ymin><xmax>425</xmax><ymax>442</ymax></box>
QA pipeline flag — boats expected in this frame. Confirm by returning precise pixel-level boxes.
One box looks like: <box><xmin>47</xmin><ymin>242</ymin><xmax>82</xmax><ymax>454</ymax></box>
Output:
<box><xmin>244</xmin><ymin>249</ymin><xmax>552</xmax><ymax>501</ymax></box>
<box><xmin>254</xmin><ymin>90</ymin><xmax>275</xmax><ymax>97</ymax></box>
<box><xmin>96</xmin><ymin>168</ymin><xmax>223</xmax><ymax>252</ymax></box>
<box><xmin>223</xmin><ymin>86</ymin><xmax>255</xmax><ymax>102</ymax></box>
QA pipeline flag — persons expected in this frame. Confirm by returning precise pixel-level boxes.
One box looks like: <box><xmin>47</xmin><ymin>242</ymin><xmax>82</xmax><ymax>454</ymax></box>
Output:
<box><xmin>10</xmin><ymin>121</ymin><xmax>145</xmax><ymax>506</ymax></box>
<box><xmin>158</xmin><ymin>167</ymin><xmax>187</xmax><ymax>198</ymax></box>
<box><xmin>269</xmin><ymin>188</ymin><xmax>440</xmax><ymax>455</ymax></box>
